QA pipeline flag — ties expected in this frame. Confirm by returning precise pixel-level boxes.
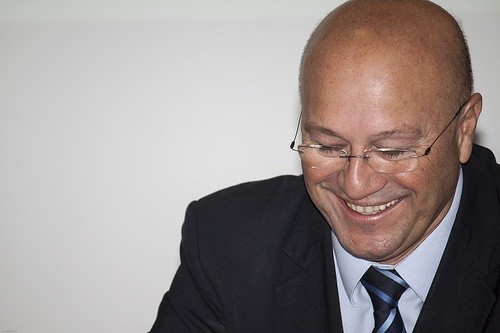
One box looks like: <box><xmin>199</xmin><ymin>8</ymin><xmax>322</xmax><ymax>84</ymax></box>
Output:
<box><xmin>360</xmin><ymin>265</ymin><xmax>410</xmax><ymax>332</ymax></box>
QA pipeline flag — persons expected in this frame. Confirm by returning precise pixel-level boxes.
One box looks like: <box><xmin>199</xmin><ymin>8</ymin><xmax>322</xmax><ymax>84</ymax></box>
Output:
<box><xmin>147</xmin><ymin>0</ymin><xmax>500</xmax><ymax>333</ymax></box>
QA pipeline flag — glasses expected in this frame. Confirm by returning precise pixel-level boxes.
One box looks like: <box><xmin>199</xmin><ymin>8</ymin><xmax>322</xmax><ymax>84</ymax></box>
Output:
<box><xmin>289</xmin><ymin>96</ymin><xmax>468</xmax><ymax>174</ymax></box>
